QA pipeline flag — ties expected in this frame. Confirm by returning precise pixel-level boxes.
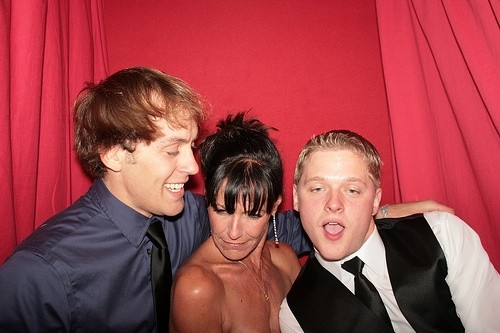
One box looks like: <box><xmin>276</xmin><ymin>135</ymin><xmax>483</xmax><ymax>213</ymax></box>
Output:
<box><xmin>146</xmin><ymin>217</ymin><xmax>172</xmax><ymax>333</ymax></box>
<box><xmin>341</xmin><ymin>255</ymin><xmax>394</xmax><ymax>333</ymax></box>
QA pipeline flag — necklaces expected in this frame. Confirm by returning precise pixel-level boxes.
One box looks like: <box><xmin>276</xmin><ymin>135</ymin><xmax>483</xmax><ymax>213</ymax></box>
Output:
<box><xmin>238</xmin><ymin>260</ymin><xmax>271</xmax><ymax>304</ymax></box>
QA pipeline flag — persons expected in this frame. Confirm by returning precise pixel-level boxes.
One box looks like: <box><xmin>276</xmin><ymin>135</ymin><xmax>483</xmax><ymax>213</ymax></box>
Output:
<box><xmin>279</xmin><ymin>130</ymin><xmax>500</xmax><ymax>333</ymax></box>
<box><xmin>170</xmin><ymin>111</ymin><xmax>301</xmax><ymax>333</ymax></box>
<box><xmin>0</xmin><ymin>65</ymin><xmax>455</xmax><ymax>333</ymax></box>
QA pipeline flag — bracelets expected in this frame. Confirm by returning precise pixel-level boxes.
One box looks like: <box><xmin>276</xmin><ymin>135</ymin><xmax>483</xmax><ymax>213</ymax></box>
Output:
<box><xmin>381</xmin><ymin>203</ymin><xmax>389</xmax><ymax>219</ymax></box>
